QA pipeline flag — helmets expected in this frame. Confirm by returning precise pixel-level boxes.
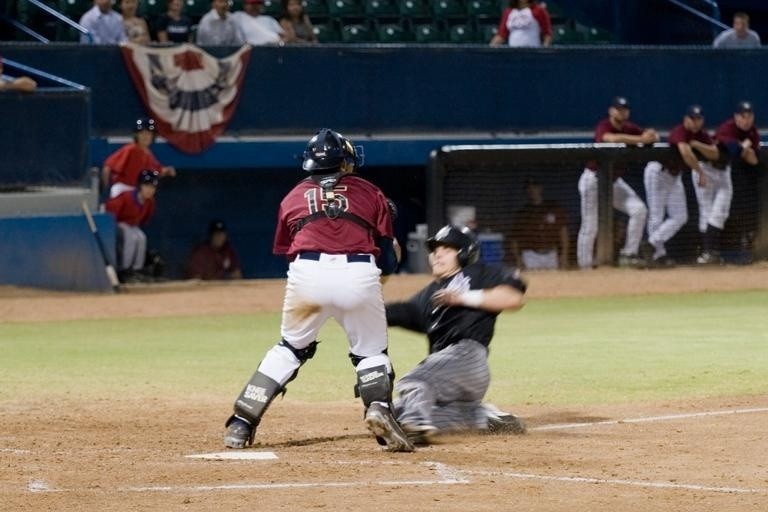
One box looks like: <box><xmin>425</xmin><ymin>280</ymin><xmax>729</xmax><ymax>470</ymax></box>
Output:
<box><xmin>302</xmin><ymin>129</ymin><xmax>365</xmax><ymax>174</ymax></box>
<box><xmin>137</xmin><ymin>169</ymin><xmax>160</xmax><ymax>187</ymax></box>
<box><xmin>425</xmin><ymin>224</ymin><xmax>481</xmax><ymax>268</ymax></box>
<box><xmin>134</xmin><ymin>119</ymin><xmax>158</xmax><ymax>134</ymax></box>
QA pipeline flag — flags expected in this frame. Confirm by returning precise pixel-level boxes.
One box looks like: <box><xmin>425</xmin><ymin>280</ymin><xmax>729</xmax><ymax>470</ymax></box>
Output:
<box><xmin>123</xmin><ymin>39</ymin><xmax>252</xmax><ymax>155</ymax></box>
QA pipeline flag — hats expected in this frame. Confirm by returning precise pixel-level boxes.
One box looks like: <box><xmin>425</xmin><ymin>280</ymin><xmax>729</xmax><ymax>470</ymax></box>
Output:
<box><xmin>685</xmin><ymin>104</ymin><xmax>704</xmax><ymax>118</ymax></box>
<box><xmin>734</xmin><ymin>101</ymin><xmax>753</xmax><ymax>113</ymax></box>
<box><xmin>611</xmin><ymin>97</ymin><xmax>630</xmax><ymax>108</ymax></box>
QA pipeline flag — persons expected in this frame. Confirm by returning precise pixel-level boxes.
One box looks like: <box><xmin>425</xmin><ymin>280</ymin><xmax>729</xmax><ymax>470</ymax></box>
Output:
<box><xmin>222</xmin><ymin>130</ymin><xmax>416</xmax><ymax>455</ymax></box>
<box><xmin>79</xmin><ymin>0</ymin><xmax>319</xmax><ymax>50</ymax></box>
<box><xmin>512</xmin><ymin>179</ymin><xmax>569</xmax><ymax>269</ymax></box>
<box><xmin>713</xmin><ymin>13</ymin><xmax>761</xmax><ymax>47</ymax></box>
<box><xmin>106</xmin><ymin>167</ymin><xmax>159</xmax><ymax>282</ymax></box>
<box><xmin>489</xmin><ymin>0</ymin><xmax>552</xmax><ymax>47</ymax></box>
<box><xmin>692</xmin><ymin>101</ymin><xmax>760</xmax><ymax>264</ymax></box>
<box><xmin>0</xmin><ymin>58</ymin><xmax>36</xmax><ymax>92</ymax></box>
<box><xmin>645</xmin><ymin>104</ymin><xmax>719</xmax><ymax>269</ymax></box>
<box><xmin>102</xmin><ymin>117</ymin><xmax>177</xmax><ymax>196</ymax></box>
<box><xmin>578</xmin><ymin>98</ymin><xmax>659</xmax><ymax>268</ymax></box>
<box><xmin>387</xmin><ymin>223</ymin><xmax>528</xmax><ymax>444</ymax></box>
<box><xmin>186</xmin><ymin>218</ymin><xmax>246</xmax><ymax>282</ymax></box>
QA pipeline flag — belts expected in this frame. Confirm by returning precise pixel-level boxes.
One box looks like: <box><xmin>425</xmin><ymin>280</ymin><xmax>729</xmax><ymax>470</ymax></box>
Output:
<box><xmin>299</xmin><ymin>251</ymin><xmax>371</xmax><ymax>262</ymax></box>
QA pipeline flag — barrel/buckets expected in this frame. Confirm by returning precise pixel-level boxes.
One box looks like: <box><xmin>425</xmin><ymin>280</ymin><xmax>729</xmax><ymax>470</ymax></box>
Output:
<box><xmin>406</xmin><ymin>234</ymin><xmax>430</xmax><ymax>272</ymax></box>
<box><xmin>476</xmin><ymin>236</ymin><xmax>505</xmax><ymax>270</ymax></box>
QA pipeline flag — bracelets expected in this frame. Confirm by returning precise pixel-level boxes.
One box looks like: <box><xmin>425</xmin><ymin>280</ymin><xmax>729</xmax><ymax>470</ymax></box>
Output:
<box><xmin>461</xmin><ymin>288</ymin><xmax>484</xmax><ymax>308</ymax></box>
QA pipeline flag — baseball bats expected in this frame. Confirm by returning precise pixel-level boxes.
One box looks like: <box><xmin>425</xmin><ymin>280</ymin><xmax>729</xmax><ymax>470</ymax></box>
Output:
<box><xmin>81</xmin><ymin>200</ymin><xmax>121</xmax><ymax>294</ymax></box>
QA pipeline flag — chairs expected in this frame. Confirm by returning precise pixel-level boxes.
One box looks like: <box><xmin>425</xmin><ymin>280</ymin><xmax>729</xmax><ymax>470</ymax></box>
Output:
<box><xmin>144</xmin><ymin>0</ymin><xmax>607</xmax><ymax>45</ymax></box>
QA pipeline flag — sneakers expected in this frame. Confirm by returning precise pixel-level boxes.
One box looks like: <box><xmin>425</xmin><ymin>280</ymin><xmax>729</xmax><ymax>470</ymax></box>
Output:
<box><xmin>224</xmin><ymin>420</ymin><xmax>251</xmax><ymax>449</ymax></box>
<box><xmin>617</xmin><ymin>245</ymin><xmax>755</xmax><ymax>269</ymax></box>
<box><xmin>478</xmin><ymin>404</ymin><xmax>526</xmax><ymax>435</ymax></box>
<box><xmin>365</xmin><ymin>402</ymin><xmax>438</xmax><ymax>451</ymax></box>
